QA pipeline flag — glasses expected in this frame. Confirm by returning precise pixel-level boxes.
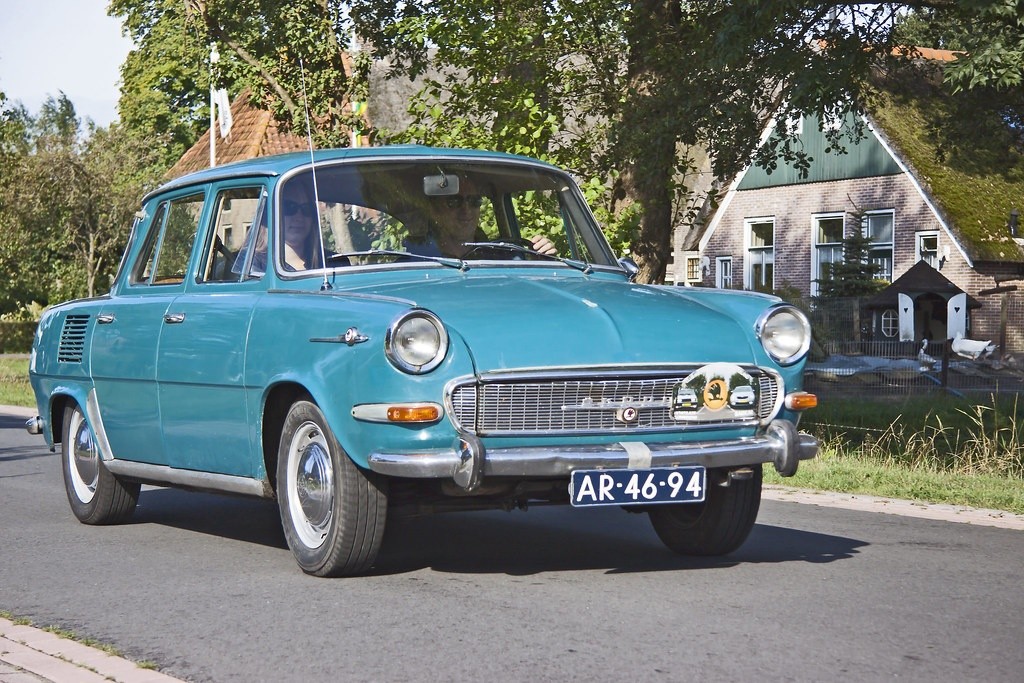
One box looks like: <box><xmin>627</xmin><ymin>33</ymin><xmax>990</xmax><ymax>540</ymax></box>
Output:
<box><xmin>440</xmin><ymin>194</ymin><xmax>483</xmax><ymax>209</ymax></box>
<box><xmin>281</xmin><ymin>200</ymin><xmax>314</xmax><ymax>218</ymax></box>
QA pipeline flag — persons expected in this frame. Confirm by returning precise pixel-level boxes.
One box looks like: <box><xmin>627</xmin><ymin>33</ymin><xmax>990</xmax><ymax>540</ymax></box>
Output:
<box><xmin>400</xmin><ymin>173</ymin><xmax>558</xmax><ymax>260</ymax></box>
<box><xmin>224</xmin><ymin>180</ymin><xmax>351</xmax><ymax>282</ymax></box>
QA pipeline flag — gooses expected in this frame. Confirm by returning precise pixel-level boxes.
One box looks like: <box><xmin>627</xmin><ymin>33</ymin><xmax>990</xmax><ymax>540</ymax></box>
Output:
<box><xmin>951</xmin><ymin>331</ymin><xmax>1000</xmax><ymax>360</ymax></box>
<box><xmin>918</xmin><ymin>339</ymin><xmax>937</xmax><ymax>372</ymax></box>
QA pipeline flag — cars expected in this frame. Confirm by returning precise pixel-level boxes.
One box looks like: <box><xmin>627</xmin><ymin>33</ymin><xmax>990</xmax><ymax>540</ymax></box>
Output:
<box><xmin>24</xmin><ymin>148</ymin><xmax>820</xmax><ymax>577</ymax></box>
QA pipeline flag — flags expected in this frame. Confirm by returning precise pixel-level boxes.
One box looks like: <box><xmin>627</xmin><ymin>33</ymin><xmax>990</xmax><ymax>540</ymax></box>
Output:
<box><xmin>213</xmin><ymin>46</ymin><xmax>233</xmax><ymax>138</ymax></box>
